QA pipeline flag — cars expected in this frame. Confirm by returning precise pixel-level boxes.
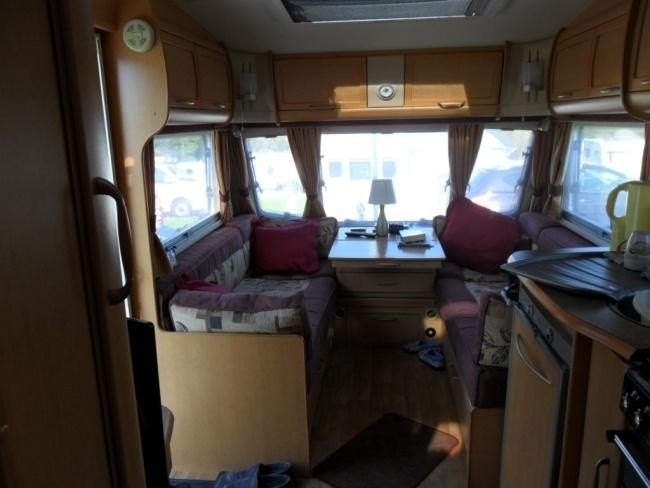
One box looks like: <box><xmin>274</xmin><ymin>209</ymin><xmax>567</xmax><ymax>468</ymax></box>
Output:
<box><xmin>155</xmin><ymin>164</ymin><xmax>210</xmax><ymax>217</ymax></box>
<box><xmin>465</xmin><ymin>164</ymin><xmax>637</xmax><ymax>224</ymax></box>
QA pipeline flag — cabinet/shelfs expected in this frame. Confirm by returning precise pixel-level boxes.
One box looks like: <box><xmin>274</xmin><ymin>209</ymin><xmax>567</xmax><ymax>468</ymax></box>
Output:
<box><xmin>110</xmin><ymin>0</ymin><xmax>234</xmax><ymax>127</ymax></box>
<box><xmin>328</xmin><ymin>225</ymin><xmax>447</xmax><ymax>351</ymax></box>
<box><xmin>500</xmin><ymin>272</ymin><xmax>648</xmax><ymax>488</ymax></box>
<box><xmin>272</xmin><ymin>46</ymin><xmax>507</xmax><ymax>124</ymax></box>
<box><xmin>549</xmin><ymin>0</ymin><xmax>648</xmax><ymax>104</ymax></box>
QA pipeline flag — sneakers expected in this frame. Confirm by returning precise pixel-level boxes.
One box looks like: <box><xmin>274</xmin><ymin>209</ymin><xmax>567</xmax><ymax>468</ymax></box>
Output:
<box><xmin>403</xmin><ymin>338</ymin><xmax>442</xmax><ymax>353</ymax></box>
<box><xmin>419</xmin><ymin>348</ymin><xmax>445</xmax><ymax>370</ymax></box>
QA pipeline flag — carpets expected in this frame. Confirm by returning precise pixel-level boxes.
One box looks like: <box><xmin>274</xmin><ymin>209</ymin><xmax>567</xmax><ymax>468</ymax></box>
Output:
<box><xmin>311</xmin><ymin>411</ymin><xmax>458</xmax><ymax>488</ymax></box>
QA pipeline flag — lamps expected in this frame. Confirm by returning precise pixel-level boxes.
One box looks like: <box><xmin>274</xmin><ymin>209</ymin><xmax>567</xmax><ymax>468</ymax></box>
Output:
<box><xmin>368</xmin><ymin>178</ymin><xmax>397</xmax><ymax>237</ymax></box>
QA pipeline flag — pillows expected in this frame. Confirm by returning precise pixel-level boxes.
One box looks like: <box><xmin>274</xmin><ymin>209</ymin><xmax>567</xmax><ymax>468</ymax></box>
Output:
<box><xmin>438</xmin><ymin>196</ymin><xmax>522</xmax><ymax>275</ymax></box>
<box><xmin>250</xmin><ymin>220</ymin><xmax>320</xmax><ymax>275</ymax></box>
<box><xmin>173</xmin><ymin>277</ymin><xmax>231</xmax><ymax>294</ymax></box>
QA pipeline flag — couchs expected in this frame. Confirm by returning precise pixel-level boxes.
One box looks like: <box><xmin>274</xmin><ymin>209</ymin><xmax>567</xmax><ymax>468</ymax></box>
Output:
<box><xmin>156</xmin><ymin>209</ymin><xmax>338</xmax><ymax>474</ymax></box>
<box><xmin>431</xmin><ymin>208</ymin><xmax>608</xmax><ymax>488</ymax></box>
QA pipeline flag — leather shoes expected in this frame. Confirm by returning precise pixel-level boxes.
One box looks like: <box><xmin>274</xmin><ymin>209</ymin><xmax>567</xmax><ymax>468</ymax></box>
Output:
<box><xmin>219</xmin><ymin>461</ymin><xmax>294</xmax><ymax>483</ymax></box>
<box><xmin>216</xmin><ymin>473</ymin><xmax>291</xmax><ymax>488</ymax></box>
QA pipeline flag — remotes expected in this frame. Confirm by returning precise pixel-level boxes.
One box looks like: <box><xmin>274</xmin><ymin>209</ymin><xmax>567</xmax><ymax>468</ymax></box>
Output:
<box><xmin>344</xmin><ymin>230</ymin><xmax>378</xmax><ymax>238</ymax></box>
